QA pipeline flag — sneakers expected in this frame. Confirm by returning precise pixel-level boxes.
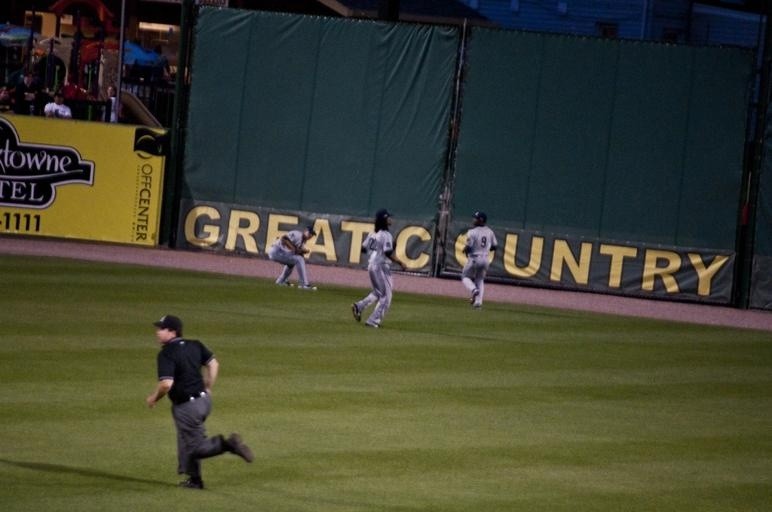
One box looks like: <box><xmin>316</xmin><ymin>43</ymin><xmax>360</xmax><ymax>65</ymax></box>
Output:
<box><xmin>284</xmin><ymin>282</ymin><xmax>293</xmax><ymax>287</ymax></box>
<box><xmin>366</xmin><ymin>322</ymin><xmax>382</xmax><ymax>328</ymax></box>
<box><xmin>470</xmin><ymin>289</ymin><xmax>479</xmax><ymax>304</ymax></box>
<box><xmin>226</xmin><ymin>431</ymin><xmax>255</xmax><ymax>465</ymax></box>
<box><xmin>298</xmin><ymin>284</ymin><xmax>317</xmax><ymax>291</ymax></box>
<box><xmin>179</xmin><ymin>479</ymin><xmax>204</xmax><ymax>489</ymax></box>
<box><xmin>351</xmin><ymin>303</ymin><xmax>362</xmax><ymax>323</ymax></box>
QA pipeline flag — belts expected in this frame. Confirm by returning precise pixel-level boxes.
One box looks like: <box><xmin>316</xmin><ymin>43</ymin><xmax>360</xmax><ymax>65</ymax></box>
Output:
<box><xmin>172</xmin><ymin>390</ymin><xmax>211</xmax><ymax>405</ymax></box>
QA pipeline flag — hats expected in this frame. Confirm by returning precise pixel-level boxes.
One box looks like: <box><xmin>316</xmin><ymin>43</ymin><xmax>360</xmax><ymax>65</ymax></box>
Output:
<box><xmin>471</xmin><ymin>209</ymin><xmax>486</xmax><ymax>225</ymax></box>
<box><xmin>306</xmin><ymin>225</ymin><xmax>317</xmax><ymax>236</ymax></box>
<box><xmin>54</xmin><ymin>89</ymin><xmax>64</xmax><ymax>95</ymax></box>
<box><xmin>375</xmin><ymin>208</ymin><xmax>394</xmax><ymax>218</ymax></box>
<box><xmin>152</xmin><ymin>314</ymin><xmax>183</xmax><ymax>329</ymax></box>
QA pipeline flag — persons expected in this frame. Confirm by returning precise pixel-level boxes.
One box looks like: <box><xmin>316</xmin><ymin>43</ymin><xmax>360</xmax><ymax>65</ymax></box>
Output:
<box><xmin>1</xmin><ymin>67</ymin><xmax>119</xmax><ymax>124</ymax></box>
<box><xmin>268</xmin><ymin>225</ymin><xmax>317</xmax><ymax>291</ymax></box>
<box><xmin>351</xmin><ymin>208</ymin><xmax>408</xmax><ymax>329</ymax></box>
<box><xmin>144</xmin><ymin>314</ymin><xmax>255</xmax><ymax>488</ymax></box>
<box><xmin>462</xmin><ymin>211</ymin><xmax>498</xmax><ymax>308</ymax></box>
